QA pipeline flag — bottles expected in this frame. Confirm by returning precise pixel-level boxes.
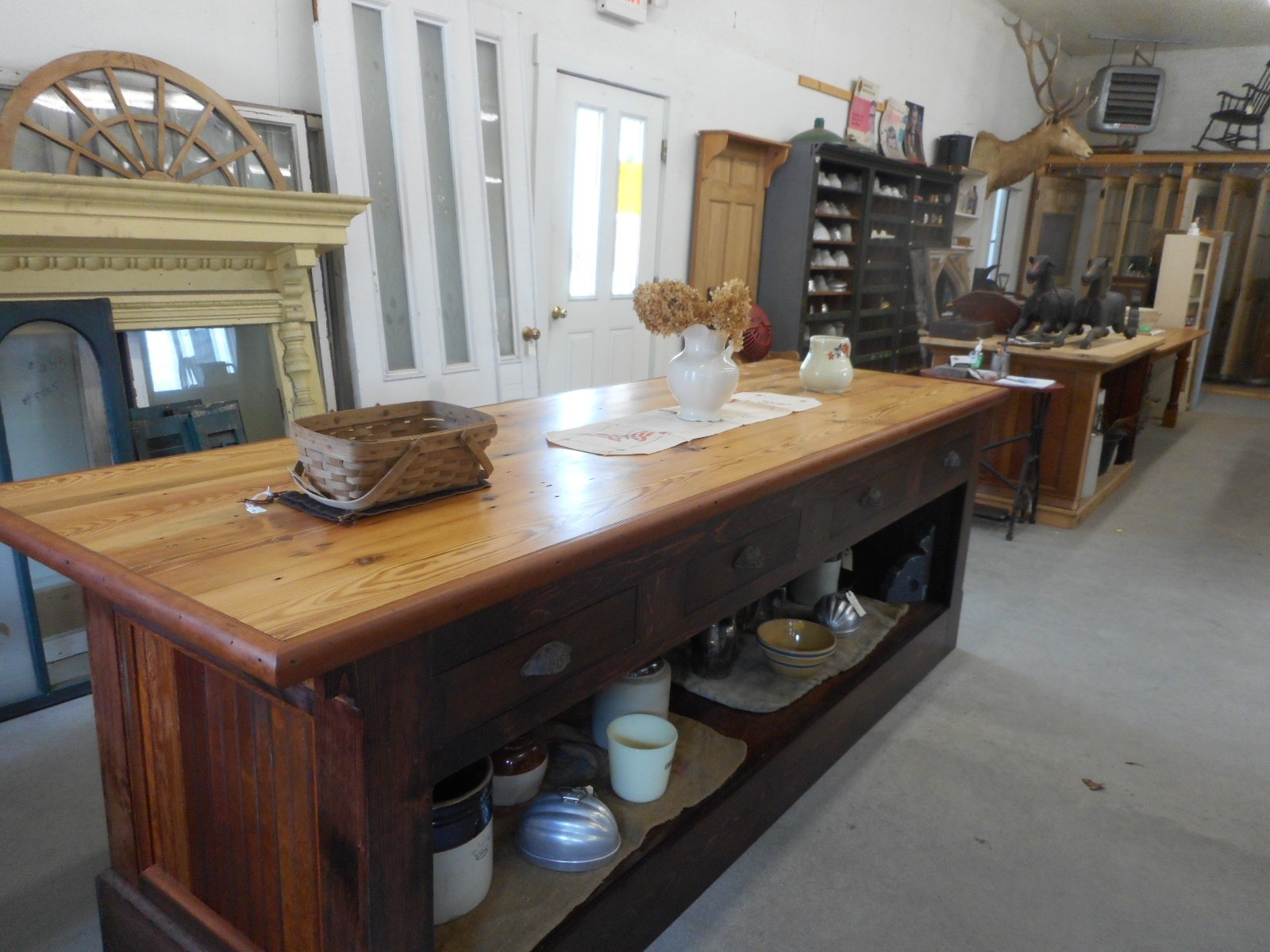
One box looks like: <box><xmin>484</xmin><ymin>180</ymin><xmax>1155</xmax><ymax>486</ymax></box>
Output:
<box><xmin>961</xmin><ymin>189</ymin><xmax>971</xmax><ymax>214</ymax></box>
<box><xmin>799</xmin><ymin>335</ymin><xmax>854</xmax><ymax>394</ymax></box>
<box><xmin>592</xmin><ymin>655</ymin><xmax>672</xmax><ymax>750</ymax></box>
<box><xmin>490</xmin><ymin>727</ymin><xmax>550</xmax><ymax>808</ymax></box>
<box><xmin>803</xmin><ymin>171</ymin><xmax>947</xmax><ymax>337</ymax></box>
<box><xmin>691</xmin><ymin>612</ymin><xmax>739</xmax><ymax>679</ymax></box>
<box><xmin>970</xmin><ymin>186</ymin><xmax>978</xmax><ymax>215</ymax></box>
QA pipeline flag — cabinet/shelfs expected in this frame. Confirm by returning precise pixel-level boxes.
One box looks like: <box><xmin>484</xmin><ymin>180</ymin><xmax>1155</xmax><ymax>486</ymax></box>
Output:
<box><xmin>1017</xmin><ymin>152</ymin><xmax>1270</xmax><ymax>421</ymax></box>
<box><xmin>0</xmin><ymin>354</ymin><xmax>1009</xmax><ymax>952</ymax></box>
<box><xmin>758</xmin><ymin>141</ymin><xmax>987</xmax><ymax>375</ymax></box>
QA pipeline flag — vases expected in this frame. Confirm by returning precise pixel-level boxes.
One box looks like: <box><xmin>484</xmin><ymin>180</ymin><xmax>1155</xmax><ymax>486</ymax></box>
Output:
<box><xmin>665</xmin><ymin>325</ymin><xmax>739</xmax><ymax>422</ymax></box>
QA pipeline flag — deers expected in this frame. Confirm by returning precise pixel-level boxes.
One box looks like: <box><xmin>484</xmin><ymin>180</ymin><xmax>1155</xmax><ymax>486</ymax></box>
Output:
<box><xmin>969</xmin><ymin>15</ymin><xmax>1098</xmax><ymax>201</ymax></box>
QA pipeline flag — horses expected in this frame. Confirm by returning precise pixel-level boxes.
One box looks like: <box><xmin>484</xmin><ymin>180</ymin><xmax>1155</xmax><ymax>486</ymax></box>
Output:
<box><xmin>1051</xmin><ymin>256</ymin><xmax>1134</xmax><ymax>349</ymax></box>
<box><xmin>1008</xmin><ymin>254</ymin><xmax>1084</xmax><ymax>342</ymax></box>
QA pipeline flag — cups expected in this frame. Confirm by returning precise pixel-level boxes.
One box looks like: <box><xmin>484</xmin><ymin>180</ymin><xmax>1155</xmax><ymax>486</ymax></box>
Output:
<box><xmin>991</xmin><ymin>352</ymin><xmax>1010</xmax><ymax>376</ymax></box>
<box><xmin>606</xmin><ymin>712</ymin><xmax>678</xmax><ymax>805</ymax></box>
<box><xmin>788</xmin><ymin>559</ymin><xmax>841</xmax><ymax>605</ymax></box>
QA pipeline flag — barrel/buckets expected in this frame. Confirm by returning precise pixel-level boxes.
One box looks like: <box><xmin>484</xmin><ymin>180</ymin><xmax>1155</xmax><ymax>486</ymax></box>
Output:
<box><xmin>939</xmin><ymin>131</ymin><xmax>974</xmax><ymax>167</ymax></box>
<box><xmin>432</xmin><ymin>752</ymin><xmax>496</xmax><ymax>926</ymax></box>
<box><xmin>1082</xmin><ymin>433</ymin><xmax>1104</xmax><ymax>498</ymax></box>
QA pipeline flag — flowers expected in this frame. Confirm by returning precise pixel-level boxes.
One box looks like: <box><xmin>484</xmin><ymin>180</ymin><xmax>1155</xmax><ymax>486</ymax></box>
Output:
<box><xmin>631</xmin><ymin>279</ymin><xmax>753</xmax><ymax>353</ymax></box>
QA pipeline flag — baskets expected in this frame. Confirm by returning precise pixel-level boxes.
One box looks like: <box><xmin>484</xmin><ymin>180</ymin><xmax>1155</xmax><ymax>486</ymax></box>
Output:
<box><xmin>291</xmin><ymin>400</ymin><xmax>498</xmax><ymax>512</ymax></box>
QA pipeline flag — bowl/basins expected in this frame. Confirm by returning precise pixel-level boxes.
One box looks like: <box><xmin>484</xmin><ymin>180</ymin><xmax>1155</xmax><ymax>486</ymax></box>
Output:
<box><xmin>1098</xmin><ymin>432</ymin><xmax>1125</xmax><ymax>477</ymax></box>
<box><xmin>756</xmin><ymin>617</ymin><xmax>837</xmax><ymax>679</ymax></box>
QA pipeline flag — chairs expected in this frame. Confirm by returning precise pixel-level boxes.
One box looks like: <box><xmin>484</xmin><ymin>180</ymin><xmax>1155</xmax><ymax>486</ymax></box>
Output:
<box><xmin>1192</xmin><ymin>60</ymin><xmax>1270</xmax><ymax>152</ymax></box>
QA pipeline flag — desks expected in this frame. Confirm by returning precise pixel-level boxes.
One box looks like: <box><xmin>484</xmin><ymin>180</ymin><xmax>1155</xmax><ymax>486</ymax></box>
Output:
<box><xmin>921</xmin><ymin>365</ymin><xmax>1065</xmax><ymax>538</ymax></box>
<box><xmin>916</xmin><ymin>335</ymin><xmax>1167</xmax><ymax>528</ymax></box>
<box><xmin>1150</xmin><ymin>328</ymin><xmax>1208</xmax><ymax>430</ymax></box>
